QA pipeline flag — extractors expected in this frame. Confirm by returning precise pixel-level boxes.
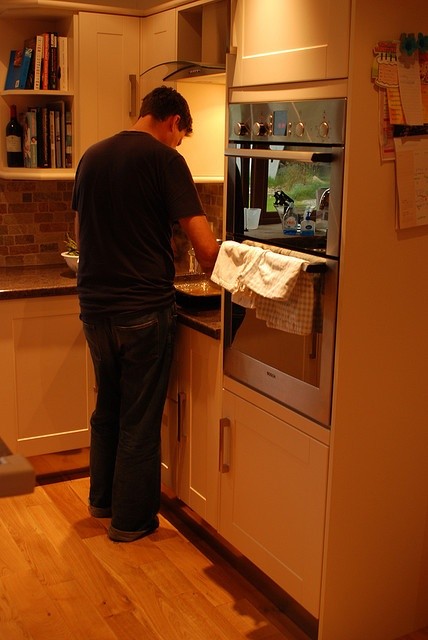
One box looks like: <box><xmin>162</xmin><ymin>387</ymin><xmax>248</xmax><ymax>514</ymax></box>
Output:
<box><xmin>163</xmin><ymin>0</ymin><xmax>230</xmax><ymax>85</ymax></box>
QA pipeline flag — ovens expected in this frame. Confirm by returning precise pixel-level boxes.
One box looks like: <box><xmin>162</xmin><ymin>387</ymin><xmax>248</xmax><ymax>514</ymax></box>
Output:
<box><xmin>221</xmin><ymin>78</ymin><xmax>349</xmax><ymax>431</ymax></box>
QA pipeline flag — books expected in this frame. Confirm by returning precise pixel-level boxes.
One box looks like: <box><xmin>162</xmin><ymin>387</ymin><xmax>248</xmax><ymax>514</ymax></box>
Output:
<box><xmin>19</xmin><ymin>112</ymin><xmax>37</xmax><ymax>168</ymax></box>
<box><xmin>48</xmin><ymin>111</ymin><xmax>56</xmax><ymax>168</ymax></box>
<box><xmin>46</xmin><ymin>100</ymin><xmax>67</xmax><ymax>168</ymax></box>
<box><xmin>41</xmin><ymin>33</ymin><xmax>50</xmax><ymax>90</ymax></box>
<box><xmin>57</xmin><ymin>36</ymin><xmax>68</xmax><ymax>91</ymax></box>
<box><xmin>41</xmin><ymin>108</ymin><xmax>49</xmax><ymax>168</ymax></box>
<box><xmin>50</xmin><ymin>32</ymin><xmax>54</xmax><ymax>90</ymax></box>
<box><xmin>28</xmin><ymin>107</ymin><xmax>43</xmax><ymax>168</ymax></box>
<box><xmin>52</xmin><ymin>35</ymin><xmax>58</xmax><ymax>89</ymax></box>
<box><xmin>65</xmin><ymin>112</ymin><xmax>72</xmax><ymax>168</ymax></box>
<box><xmin>24</xmin><ymin>36</ymin><xmax>44</xmax><ymax>90</ymax></box>
<box><xmin>5</xmin><ymin>48</ymin><xmax>33</xmax><ymax>89</ymax></box>
<box><xmin>54</xmin><ymin>111</ymin><xmax>62</xmax><ymax>168</ymax></box>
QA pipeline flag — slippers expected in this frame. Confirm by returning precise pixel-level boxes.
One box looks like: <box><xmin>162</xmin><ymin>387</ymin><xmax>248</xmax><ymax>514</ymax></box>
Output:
<box><xmin>106</xmin><ymin>518</ymin><xmax>157</xmax><ymax>542</ymax></box>
<box><xmin>87</xmin><ymin>504</ymin><xmax>110</xmax><ymax>516</ymax></box>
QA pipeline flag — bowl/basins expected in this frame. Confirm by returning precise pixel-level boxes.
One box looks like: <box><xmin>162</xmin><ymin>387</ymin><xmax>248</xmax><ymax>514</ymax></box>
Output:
<box><xmin>60</xmin><ymin>251</ymin><xmax>80</xmax><ymax>274</ymax></box>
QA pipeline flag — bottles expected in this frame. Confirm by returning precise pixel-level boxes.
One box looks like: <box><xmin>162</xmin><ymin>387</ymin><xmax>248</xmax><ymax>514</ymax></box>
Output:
<box><xmin>5</xmin><ymin>104</ymin><xmax>25</xmax><ymax>168</ymax></box>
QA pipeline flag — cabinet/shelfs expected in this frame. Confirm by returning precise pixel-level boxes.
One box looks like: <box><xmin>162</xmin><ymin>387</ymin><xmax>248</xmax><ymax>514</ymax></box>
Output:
<box><xmin>0</xmin><ymin>1</ymin><xmax>76</xmax><ymax>180</ymax></box>
<box><xmin>138</xmin><ymin>1</ymin><xmax>201</xmax><ymax>80</ymax></box>
<box><xmin>216</xmin><ymin>377</ymin><xmax>327</xmax><ymax>621</ymax></box>
<box><xmin>173</xmin><ymin>73</ymin><xmax>225</xmax><ymax>187</ymax></box>
<box><xmin>158</xmin><ymin>323</ymin><xmax>219</xmax><ymax>532</ymax></box>
<box><xmin>76</xmin><ymin>3</ymin><xmax>141</xmax><ymax>153</ymax></box>
<box><xmin>1</xmin><ymin>298</ymin><xmax>98</xmax><ymax>480</ymax></box>
<box><xmin>226</xmin><ymin>0</ymin><xmax>353</xmax><ymax>89</ymax></box>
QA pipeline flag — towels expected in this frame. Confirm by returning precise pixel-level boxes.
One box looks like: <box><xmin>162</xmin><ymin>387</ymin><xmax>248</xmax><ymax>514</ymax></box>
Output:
<box><xmin>238</xmin><ymin>248</ymin><xmax>315</xmax><ymax>337</ymax></box>
<box><xmin>211</xmin><ymin>241</ymin><xmax>264</xmax><ymax>310</ymax></box>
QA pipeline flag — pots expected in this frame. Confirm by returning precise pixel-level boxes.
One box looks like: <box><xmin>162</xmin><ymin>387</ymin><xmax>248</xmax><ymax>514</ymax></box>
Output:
<box><xmin>170</xmin><ymin>273</ymin><xmax>222</xmax><ymax>312</ymax></box>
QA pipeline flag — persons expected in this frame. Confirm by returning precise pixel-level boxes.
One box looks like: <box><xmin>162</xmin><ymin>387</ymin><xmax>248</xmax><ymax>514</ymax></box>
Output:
<box><xmin>70</xmin><ymin>85</ymin><xmax>220</xmax><ymax>543</ymax></box>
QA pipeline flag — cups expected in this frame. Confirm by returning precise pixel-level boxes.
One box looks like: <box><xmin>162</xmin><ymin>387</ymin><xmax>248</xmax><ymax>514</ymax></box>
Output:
<box><xmin>247</xmin><ymin>207</ymin><xmax>262</xmax><ymax>230</ymax></box>
<box><xmin>244</xmin><ymin>208</ymin><xmax>249</xmax><ymax>230</ymax></box>
